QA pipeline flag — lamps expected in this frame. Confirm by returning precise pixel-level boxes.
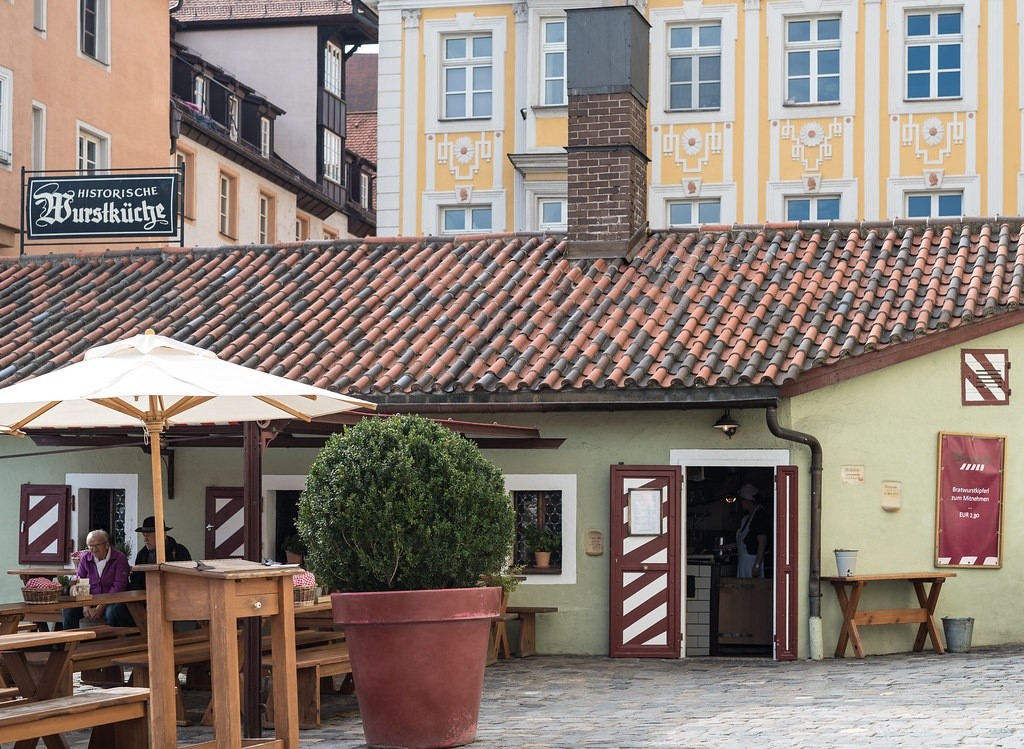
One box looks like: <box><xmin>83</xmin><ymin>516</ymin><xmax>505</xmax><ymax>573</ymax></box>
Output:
<box><xmin>712</xmin><ymin>409</ymin><xmax>740</xmax><ymax>438</ymax></box>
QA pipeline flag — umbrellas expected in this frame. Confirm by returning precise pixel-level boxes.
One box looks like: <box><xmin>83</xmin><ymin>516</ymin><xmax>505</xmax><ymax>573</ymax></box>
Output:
<box><xmin>0</xmin><ymin>329</ymin><xmax>378</xmax><ymax>566</ymax></box>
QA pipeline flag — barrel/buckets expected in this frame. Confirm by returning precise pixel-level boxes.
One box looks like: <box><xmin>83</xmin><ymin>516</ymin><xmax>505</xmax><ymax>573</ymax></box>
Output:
<box><xmin>941</xmin><ymin>616</ymin><xmax>975</xmax><ymax>653</ymax></box>
<box><xmin>834</xmin><ymin>551</ymin><xmax>857</xmax><ymax>576</ymax></box>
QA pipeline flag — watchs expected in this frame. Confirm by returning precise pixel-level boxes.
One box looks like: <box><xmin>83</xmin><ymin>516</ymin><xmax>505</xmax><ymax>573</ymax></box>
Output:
<box><xmin>753</xmin><ymin>564</ymin><xmax>760</xmax><ymax>568</ymax></box>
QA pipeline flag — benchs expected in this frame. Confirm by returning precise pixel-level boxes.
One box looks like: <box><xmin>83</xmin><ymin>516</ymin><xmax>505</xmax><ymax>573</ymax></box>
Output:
<box><xmin>0</xmin><ymin>622</ymin><xmax>353</xmax><ymax>749</ymax></box>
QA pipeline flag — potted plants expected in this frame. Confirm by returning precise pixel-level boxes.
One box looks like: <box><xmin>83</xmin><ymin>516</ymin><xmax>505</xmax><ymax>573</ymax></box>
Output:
<box><xmin>525</xmin><ymin>526</ymin><xmax>560</xmax><ymax>568</ymax></box>
<box><xmin>294</xmin><ymin>414</ymin><xmax>527</xmax><ymax>749</ymax></box>
<box><xmin>283</xmin><ymin>534</ymin><xmax>307</xmax><ymax>566</ymax></box>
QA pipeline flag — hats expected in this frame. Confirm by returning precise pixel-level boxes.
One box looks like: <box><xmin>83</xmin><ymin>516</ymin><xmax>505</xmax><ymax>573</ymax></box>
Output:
<box><xmin>135</xmin><ymin>517</ymin><xmax>172</xmax><ymax>532</ymax></box>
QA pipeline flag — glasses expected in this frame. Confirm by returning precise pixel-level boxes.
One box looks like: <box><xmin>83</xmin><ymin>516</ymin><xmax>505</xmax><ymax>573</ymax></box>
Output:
<box><xmin>88</xmin><ymin>542</ymin><xmax>105</xmax><ymax>548</ymax></box>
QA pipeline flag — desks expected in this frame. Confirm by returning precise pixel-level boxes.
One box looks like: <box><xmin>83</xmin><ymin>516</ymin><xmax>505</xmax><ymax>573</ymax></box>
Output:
<box><xmin>818</xmin><ymin>572</ymin><xmax>957</xmax><ymax>659</ymax></box>
<box><xmin>200</xmin><ymin>595</ymin><xmax>333</xmax><ymax>724</ymax></box>
<box><xmin>0</xmin><ymin>588</ymin><xmax>147</xmax><ymax>637</ymax></box>
<box><xmin>0</xmin><ymin>631</ymin><xmax>97</xmax><ymax>749</ymax></box>
<box><xmin>7</xmin><ymin>568</ymin><xmax>76</xmax><ymax>585</ymax></box>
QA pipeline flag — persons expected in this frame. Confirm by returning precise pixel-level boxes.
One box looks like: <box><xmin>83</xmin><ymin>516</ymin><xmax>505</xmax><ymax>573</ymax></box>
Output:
<box><xmin>718</xmin><ymin>483</ymin><xmax>771</xmax><ymax>579</ymax></box>
<box><xmin>63</xmin><ymin>514</ymin><xmax>191</xmax><ymax>637</ymax></box>
